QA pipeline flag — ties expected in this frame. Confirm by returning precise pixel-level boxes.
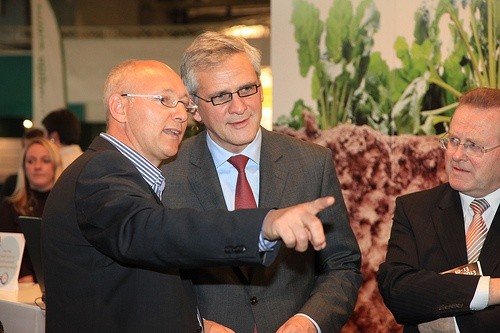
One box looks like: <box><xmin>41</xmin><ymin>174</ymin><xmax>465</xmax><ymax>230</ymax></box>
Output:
<box><xmin>227</xmin><ymin>155</ymin><xmax>258</xmax><ymax>333</ymax></box>
<box><xmin>465</xmin><ymin>198</ymin><xmax>490</xmax><ymax>265</ymax></box>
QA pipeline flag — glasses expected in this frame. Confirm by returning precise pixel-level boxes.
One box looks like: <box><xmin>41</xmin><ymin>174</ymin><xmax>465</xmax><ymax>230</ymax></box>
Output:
<box><xmin>440</xmin><ymin>131</ymin><xmax>500</xmax><ymax>157</ymax></box>
<box><xmin>193</xmin><ymin>80</ymin><xmax>261</xmax><ymax>106</ymax></box>
<box><xmin>121</xmin><ymin>94</ymin><xmax>198</xmax><ymax>113</ymax></box>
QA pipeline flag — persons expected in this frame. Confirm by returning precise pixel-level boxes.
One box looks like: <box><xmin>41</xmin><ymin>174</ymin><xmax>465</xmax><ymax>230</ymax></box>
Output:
<box><xmin>156</xmin><ymin>30</ymin><xmax>363</xmax><ymax>333</ymax></box>
<box><xmin>39</xmin><ymin>60</ymin><xmax>336</xmax><ymax>333</ymax></box>
<box><xmin>0</xmin><ymin>108</ymin><xmax>85</xmax><ymax>282</ymax></box>
<box><xmin>376</xmin><ymin>87</ymin><xmax>500</xmax><ymax>333</ymax></box>
